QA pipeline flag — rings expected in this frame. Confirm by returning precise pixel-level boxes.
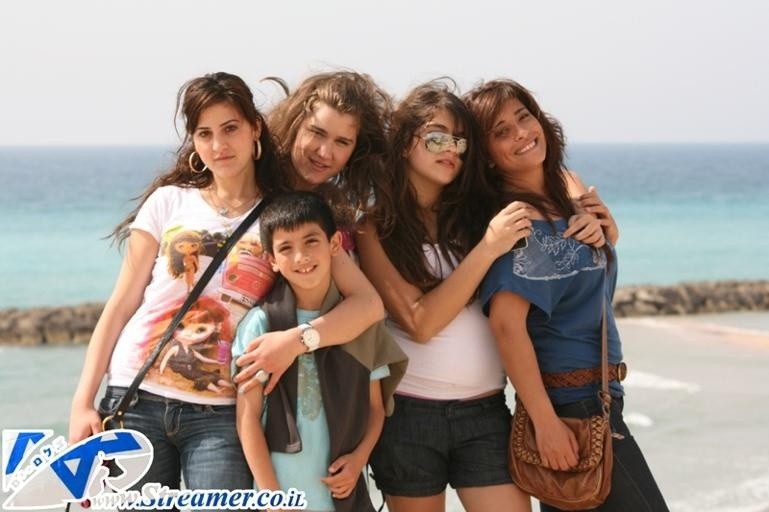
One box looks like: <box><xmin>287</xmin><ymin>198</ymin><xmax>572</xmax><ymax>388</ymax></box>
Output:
<box><xmin>255</xmin><ymin>370</ymin><xmax>268</xmax><ymax>383</ymax></box>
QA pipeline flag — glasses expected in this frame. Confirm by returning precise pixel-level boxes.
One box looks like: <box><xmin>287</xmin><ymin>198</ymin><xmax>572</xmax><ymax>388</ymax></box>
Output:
<box><xmin>414</xmin><ymin>130</ymin><xmax>469</xmax><ymax>154</ymax></box>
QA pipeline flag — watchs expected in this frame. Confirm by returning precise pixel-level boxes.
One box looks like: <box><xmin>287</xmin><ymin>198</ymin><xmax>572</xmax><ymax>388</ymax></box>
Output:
<box><xmin>298</xmin><ymin>323</ymin><xmax>321</xmax><ymax>353</ymax></box>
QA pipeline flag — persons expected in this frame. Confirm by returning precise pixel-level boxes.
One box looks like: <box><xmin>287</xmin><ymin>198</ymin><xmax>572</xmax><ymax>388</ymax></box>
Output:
<box><xmin>462</xmin><ymin>80</ymin><xmax>668</xmax><ymax>512</ymax></box>
<box><xmin>259</xmin><ymin>72</ymin><xmax>398</xmax><ymax>270</ymax></box>
<box><xmin>68</xmin><ymin>73</ymin><xmax>385</xmax><ymax>512</ymax></box>
<box><xmin>353</xmin><ymin>76</ymin><xmax>606</xmax><ymax>512</ymax></box>
<box><xmin>167</xmin><ymin>230</ymin><xmax>203</xmax><ymax>295</ymax></box>
<box><xmin>160</xmin><ymin>296</ymin><xmax>237</xmax><ymax>393</ymax></box>
<box><xmin>232</xmin><ymin>192</ymin><xmax>390</xmax><ymax>512</ymax></box>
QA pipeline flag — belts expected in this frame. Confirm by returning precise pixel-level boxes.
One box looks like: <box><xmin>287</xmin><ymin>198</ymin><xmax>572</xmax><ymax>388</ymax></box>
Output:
<box><xmin>540</xmin><ymin>360</ymin><xmax>626</xmax><ymax>389</ymax></box>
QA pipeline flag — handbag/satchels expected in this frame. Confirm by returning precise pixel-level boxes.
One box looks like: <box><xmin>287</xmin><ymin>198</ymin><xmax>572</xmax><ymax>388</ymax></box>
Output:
<box><xmin>508</xmin><ymin>401</ymin><xmax>614</xmax><ymax>511</ymax></box>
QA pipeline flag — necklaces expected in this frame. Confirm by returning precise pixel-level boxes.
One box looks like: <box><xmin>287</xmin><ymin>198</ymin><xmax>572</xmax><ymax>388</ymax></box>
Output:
<box><xmin>207</xmin><ymin>183</ymin><xmax>262</xmax><ymax>216</ymax></box>
